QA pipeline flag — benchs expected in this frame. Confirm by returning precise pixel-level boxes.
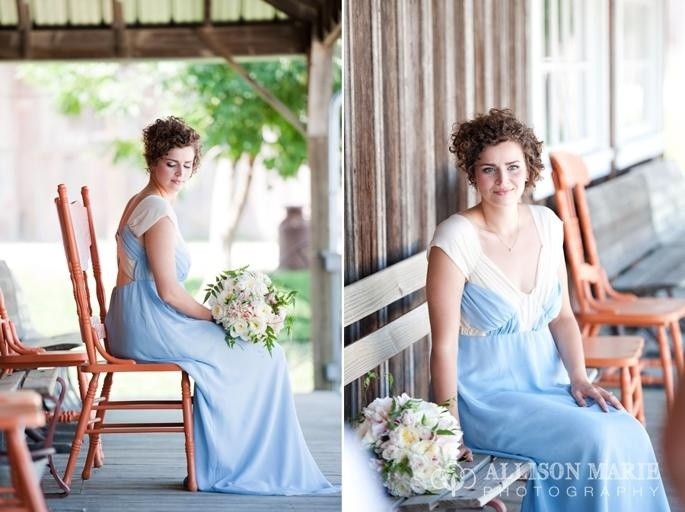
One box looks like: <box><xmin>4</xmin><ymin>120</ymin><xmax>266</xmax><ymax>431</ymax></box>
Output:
<box><xmin>344</xmin><ymin>252</ymin><xmax>534</xmax><ymax>511</ymax></box>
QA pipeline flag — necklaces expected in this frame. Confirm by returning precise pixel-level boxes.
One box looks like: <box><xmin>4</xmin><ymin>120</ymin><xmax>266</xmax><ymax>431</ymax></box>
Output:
<box><xmin>492</xmin><ymin>219</ymin><xmax>521</xmax><ymax>254</ymax></box>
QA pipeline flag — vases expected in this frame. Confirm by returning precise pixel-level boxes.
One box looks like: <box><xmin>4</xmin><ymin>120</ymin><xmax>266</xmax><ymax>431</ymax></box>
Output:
<box><xmin>278</xmin><ymin>206</ymin><xmax>311</xmax><ymax>269</ymax></box>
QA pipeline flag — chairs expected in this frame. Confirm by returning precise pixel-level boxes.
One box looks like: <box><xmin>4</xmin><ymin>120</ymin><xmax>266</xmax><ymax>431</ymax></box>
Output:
<box><xmin>547</xmin><ymin>152</ymin><xmax>685</xmax><ymax>430</ymax></box>
<box><xmin>1</xmin><ymin>181</ymin><xmax>196</xmax><ymax>511</ymax></box>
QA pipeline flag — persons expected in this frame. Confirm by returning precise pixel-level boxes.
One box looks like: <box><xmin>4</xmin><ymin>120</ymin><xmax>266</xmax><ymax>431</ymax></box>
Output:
<box><xmin>104</xmin><ymin>116</ymin><xmax>341</xmax><ymax>497</ymax></box>
<box><xmin>426</xmin><ymin>107</ymin><xmax>672</xmax><ymax>512</ymax></box>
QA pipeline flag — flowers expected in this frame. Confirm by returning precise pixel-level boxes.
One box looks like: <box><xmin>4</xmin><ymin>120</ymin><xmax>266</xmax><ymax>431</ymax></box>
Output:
<box><xmin>350</xmin><ymin>391</ymin><xmax>462</xmax><ymax>495</ymax></box>
<box><xmin>203</xmin><ymin>271</ymin><xmax>299</xmax><ymax>344</ymax></box>
